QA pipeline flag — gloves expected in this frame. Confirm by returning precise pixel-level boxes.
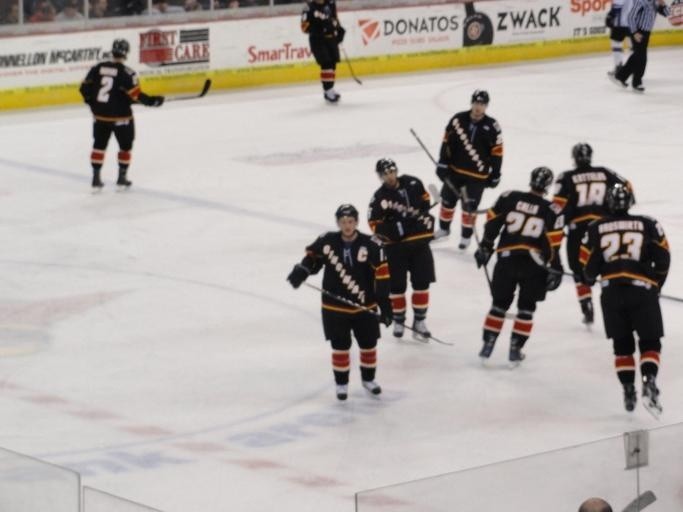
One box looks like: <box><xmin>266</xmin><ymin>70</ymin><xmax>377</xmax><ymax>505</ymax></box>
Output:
<box><xmin>547</xmin><ymin>264</ymin><xmax>562</xmax><ymax>290</ymax></box>
<box><xmin>150</xmin><ymin>94</ymin><xmax>163</xmax><ymax>106</ymax></box>
<box><xmin>581</xmin><ymin>268</ymin><xmax>595</xmax><ymax>288</ymax></box>
<box><xmin>475</xmin><ymin>240</ymin><xmax>494</xmax><ymax>269</ymax></box>
<box><xmin>374</xmin><ymin>297</ymin><xmax>392</xmax><ymax>327</ymax></box>
<box><xmin>288</xmin><ymin>266</ymin><xmax>307</xmax><ymax>289</ymax></box>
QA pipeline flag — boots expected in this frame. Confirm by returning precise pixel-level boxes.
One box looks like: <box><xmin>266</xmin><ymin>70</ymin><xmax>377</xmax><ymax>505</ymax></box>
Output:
<box><xmin>92</xmin><ymin>175</ymin><xmax>103</xmax><ymax>187</ymax></box>
<box><xmin>118</xmin><ymin>169</ymin><xmax>131</xmax><ymax>185</ymax></box>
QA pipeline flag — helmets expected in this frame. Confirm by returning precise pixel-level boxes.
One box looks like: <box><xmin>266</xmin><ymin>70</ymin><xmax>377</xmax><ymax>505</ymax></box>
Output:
<box><xmin>471</xmin><ymin>89</ymin><xmax>490</xmax><ymax>106</ymax></box>
<box><xmin>112</xmin><ymin>38</ymin><xmax>130</xmax><ymax>57</ymax></box>
<box><xmin>375</xmin><ymin>144</ymin><xmax>632</xmax><ymax>208</ymax></box>
<box><xmin>335</xmin><ymin>201</ymin><xmax>358</xmax><ymax>216</ymax></box>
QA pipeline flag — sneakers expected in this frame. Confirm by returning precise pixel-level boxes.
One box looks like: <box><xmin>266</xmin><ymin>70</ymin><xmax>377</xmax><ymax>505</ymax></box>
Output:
<box><xmin>624</xmin><ymin>384</ymin><xmax>636</xmax><ymax>408</ymax></box>
<box><xmin>640</xmin><ymin>376</ymin><xmax>658</xmax><ymax>407</ymax></box>
<box><xmin>479</xmin><ymin>336</ymin><xmax>495</xmax><ymax>359</ymax></box>
<box><xmin>392</xmin><ymin>317</ymin><xmax>407</xmax><ymax>337</ymax></box>
<box><xmin>361</xmin><ymin>379</ymin><xmax>382</xmax><ymax>398</ymax></box>
<box><xmin>635</xmin><ymin>83</ymin><xmax>643</xmax><ymax>90</ymax></box>
<box><xmin>431</xmin><ymin>231</ymin><xmax>450</xmax><ymax>241</ymax></box>
<box><xmin>509</xmin><ymin>339</ymin><xmax>525</xmax><ymax>368</ymax></box>
<box><xmin>338</xmin><ymin>382</ymin><xmax>348</xmax><ymax>402</ymax></box>
<box><xmin>324</xmin><ymin>89</ymin><xmax>338</xmax><ymax>101</ymax></box>
<box><xmin>459</xmin><ymin>239</ymin><xmax>470</xmax><ymax>251</ymax></box>
<box><xmin>581</xmin><ymin>302</ymin><xmax>593</xmax><ymax>330</ymax></box>
<box><xmin>413</xmin><ymin>315</ymin><xmax>431</xmax><ymax>343</ymax></box>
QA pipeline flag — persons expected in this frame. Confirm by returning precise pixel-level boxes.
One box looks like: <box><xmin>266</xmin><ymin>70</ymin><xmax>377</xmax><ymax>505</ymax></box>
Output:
<box><xmin>577</xmin><ymin>180</ymin><xmax>671</xmax><ymax>411</ymax></box>
<box><xmin>366</xmin><ymin>157</ymin><xmax>436</xmax><ymax>337</ymax></box>
<box><xmin>301</xmin><ymin>0</ymin><xmax>346</xmax><ymax>102</ymax></box>
<box><xmin>474</xmin><ymin>165</ymin><xmax>565</xmax><ymax>364</ymax></box>
<box><xmin>552</xmin><ymin>141</ymin><xmax>637</xmax><ymax>322</ymax></box>
<box><xmin>577</xmin><ymin>496</ymin><xmax>613</xmax><ymax>512</ymax></box>
<box><xmin>613</xmin><ymin>0</ymin><xmax>670</xmax><ymax>92</ymax></box>
<box><xmin>604</xmin><ymin>0</ymin><xmax>635</xmax><ymax>77</ymax></box>
<box><xmin>0</xmin><ymin>1</ymin><xmax>242</xmax><ymax>24</ymax></box>
<box><xmin>435</xmin><ymin>89</ymin><xmax>503</xmax><ymax>247</ymax></box>
<box><xmin>80</xmin><ymin>39</ymin><xmax>164</xmax><ymax>188</ymax></box>
<box><xmin>287</xmin><ymin>203</ymin><xmax>394</xmax><ymax>401</ymax></box>
